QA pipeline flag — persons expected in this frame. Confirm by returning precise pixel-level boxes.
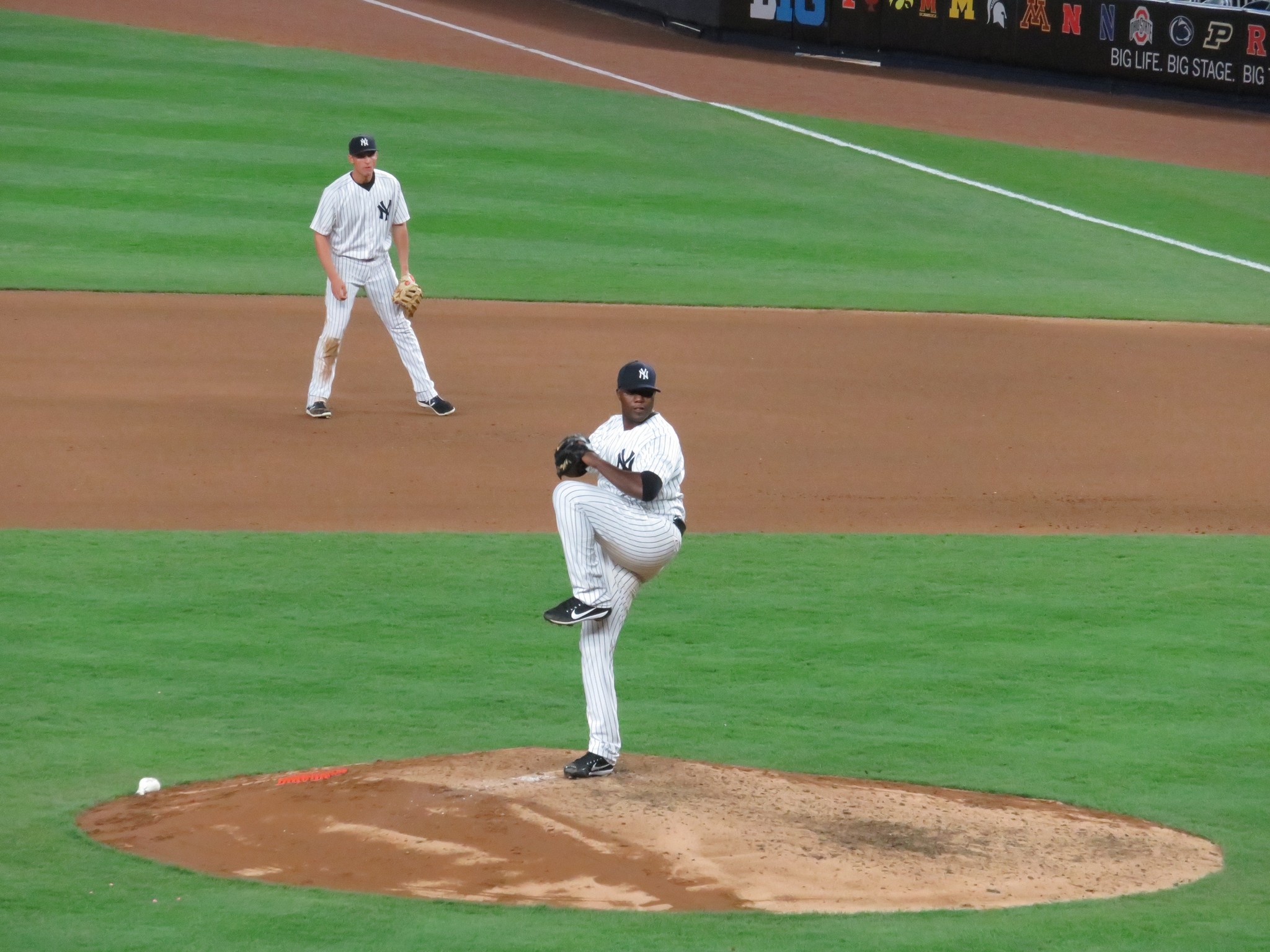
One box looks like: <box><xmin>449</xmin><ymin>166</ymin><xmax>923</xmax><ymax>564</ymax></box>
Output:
<box><xmin>544</xmin><ymin>360</ymin><xmax>686</xmax><ymax>779</ymax></box>
<box><xmin>305</xmin><ymin>138</ymin><xmax>455</xmax><ymax>415</ymax></box>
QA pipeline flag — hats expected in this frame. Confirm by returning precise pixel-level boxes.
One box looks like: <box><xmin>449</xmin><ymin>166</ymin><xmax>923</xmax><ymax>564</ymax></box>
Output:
<box><xmin>618</xmin><ymin>359</ymin><xmax>661</xmax><ymax>392</ymax></box>
<box><xmin>348</xmin><ymin>135</ymin><xmax>379</xmax><ymax>155</ymax></box>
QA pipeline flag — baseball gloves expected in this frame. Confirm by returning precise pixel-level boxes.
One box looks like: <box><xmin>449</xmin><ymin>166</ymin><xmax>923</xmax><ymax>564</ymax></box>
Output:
<box><xmin>554</xmin><ymin>433</ymin><xmax>592</xmax><ymax>480</ymax></box>
<box><xmin>392</xmin><ymin>275</ymin><xmax>423</xmax><ymax>318</ymax></box>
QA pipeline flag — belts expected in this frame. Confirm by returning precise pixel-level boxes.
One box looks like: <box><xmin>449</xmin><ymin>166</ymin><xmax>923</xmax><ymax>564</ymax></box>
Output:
<box><xmin>675</xmin><ymin>518</ymin><xmax>687</xmax><ymax>536</ymax></box>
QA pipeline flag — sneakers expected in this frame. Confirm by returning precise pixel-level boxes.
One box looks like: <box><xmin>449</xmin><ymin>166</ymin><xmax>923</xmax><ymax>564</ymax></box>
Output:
<box><xmin>306</xmin><ymin>402</ymin><xmax>332</xmax><ymax>417</ymax></box>
<box><xmin>562</xmin><ymin>753</ymin><xmax>614</xmax><ymax>778</ymax></box>
<box><xmin>544</xmin><ymin>596</ymin><xmax>612</xmax><ymax>626</ymax></box>
<box><xmin>416</xmin><ymin>395</ymin><xmax>455</xmax><ymax>416</ymax></box>
